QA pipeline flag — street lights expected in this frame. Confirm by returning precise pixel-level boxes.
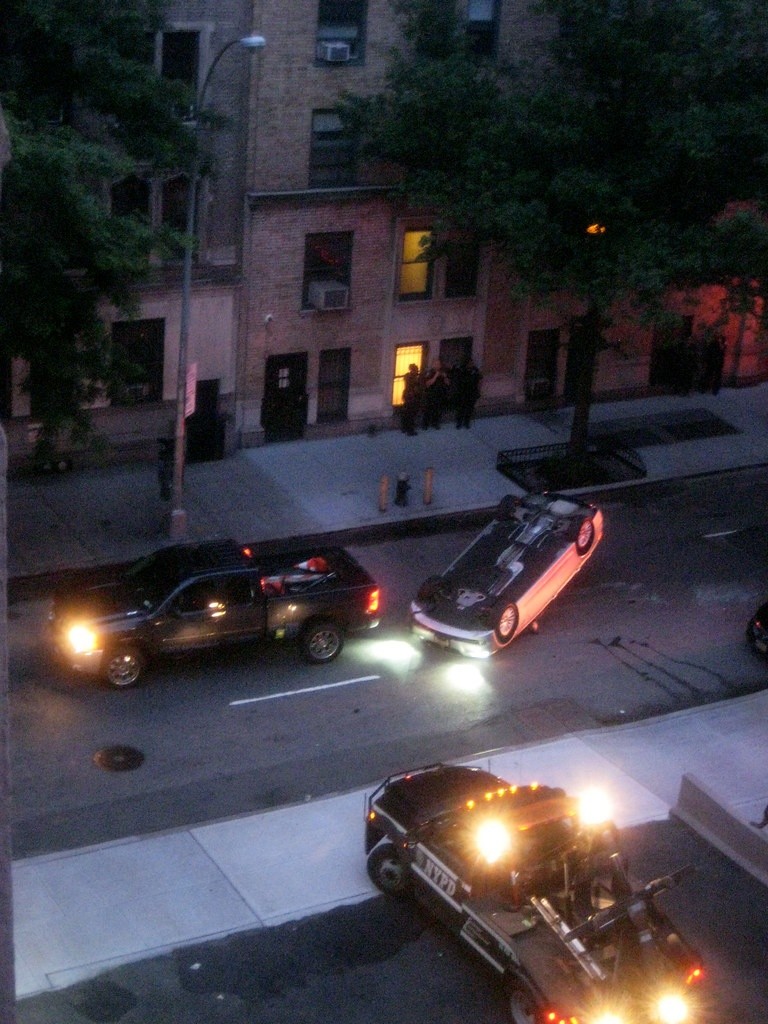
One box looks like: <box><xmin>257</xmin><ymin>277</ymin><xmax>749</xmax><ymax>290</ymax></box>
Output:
<box><xmin>161</xmin><ymin>32</ymin><xmax>268</xmax><ymax>542</ymax></box>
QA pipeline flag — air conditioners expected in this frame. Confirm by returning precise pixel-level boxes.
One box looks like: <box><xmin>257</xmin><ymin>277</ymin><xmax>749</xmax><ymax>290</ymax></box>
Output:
<box><xmin>308</xmin><ymin>282</ymin><xmax>349</xmax><ymax>311</ymax></box>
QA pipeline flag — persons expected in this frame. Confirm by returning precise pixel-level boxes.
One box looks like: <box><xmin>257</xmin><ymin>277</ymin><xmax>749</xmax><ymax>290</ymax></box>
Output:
<box><xmin>702</xmin><ymin>332</ymin><xmax>728</xmax><ymax>397</ymax></box>
<box><xmin>401</xmin><ymin>365</ymin><xmax>422</xmax><ymax>437</ymax></box>
<box><xmin>667</xmin><ymin>335</ymin><xmax>698</xmax><ymax>397</ymax></box>
<box><xmin>424</xmin><ymin>360</ymin><xmax>449</xmax><ymax>431</ymax></box>
<box><xmin>457</xmin><ymin>358</ymin><xmax>480</xmax><ymax>429</ymax></box>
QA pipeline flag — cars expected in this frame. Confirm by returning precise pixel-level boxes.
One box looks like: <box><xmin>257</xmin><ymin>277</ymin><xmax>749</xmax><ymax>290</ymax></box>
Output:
<box><xmin>407</xmin><ymin>488</ymin><xmax>612</xmax><ymax>659</ymax></box>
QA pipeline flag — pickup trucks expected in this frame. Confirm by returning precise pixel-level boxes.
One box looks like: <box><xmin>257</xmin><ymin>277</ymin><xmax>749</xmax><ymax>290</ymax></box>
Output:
<box><xmin>38</xmin><ymin>538</ymin><xmax>383</xmax><ymax>690</ymax></box>
<box><xmin>365</xmin><ymin>764</ymin><xmax>719</xmax><ymax>1024</ymax></box>
